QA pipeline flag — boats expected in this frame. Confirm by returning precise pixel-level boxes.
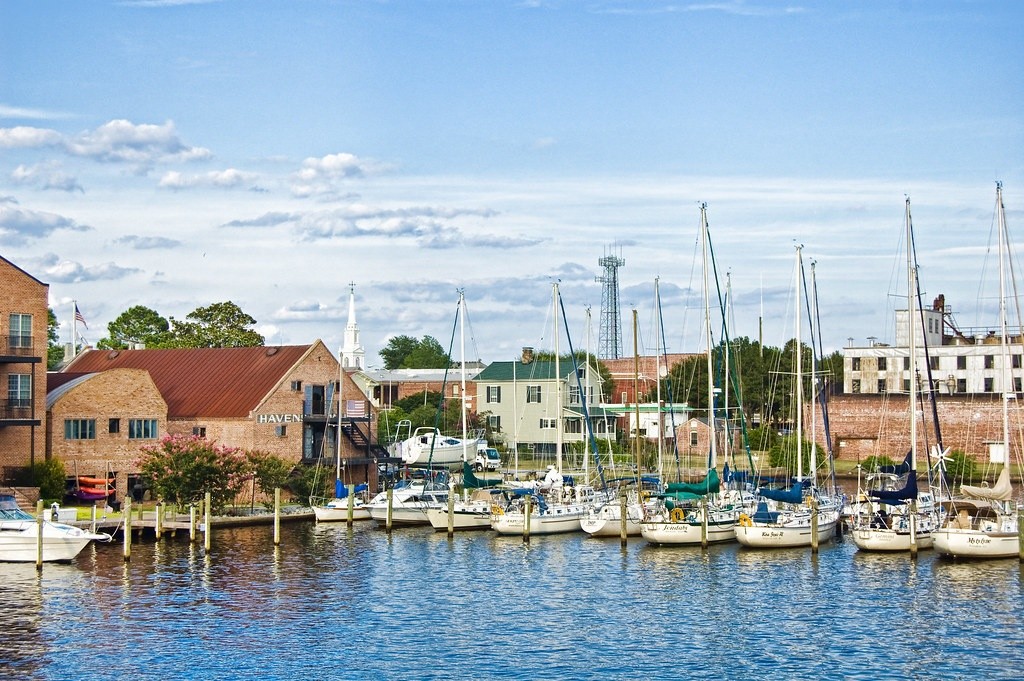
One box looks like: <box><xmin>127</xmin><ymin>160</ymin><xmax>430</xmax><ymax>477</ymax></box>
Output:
<box><xmin>0</xmin><ymin>493</ymin><xmax>112</xmax><ymax>563</ymax></box>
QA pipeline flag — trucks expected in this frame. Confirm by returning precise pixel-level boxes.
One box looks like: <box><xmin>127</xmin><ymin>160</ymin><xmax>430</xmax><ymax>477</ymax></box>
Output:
<box><xmin>475</xmin><ymin>439</ymin><xmax>502</xmax><ymax>472</ymax></box>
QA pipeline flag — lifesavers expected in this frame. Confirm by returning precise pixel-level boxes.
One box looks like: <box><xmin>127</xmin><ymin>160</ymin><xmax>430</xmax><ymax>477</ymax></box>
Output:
<box><xmin>670</xmin><ymin>508</ymin><xmax>684</xmax><ymax>523</ymax></box>
<box><xmin>490</xmin><ymin>504</ymin><xmax>504</xmax><ymax>516</ymax></box>
<box><xmin>740</xmin><ymin>513</ymin><xmax>752</xmax><ymax>526</ymax></box>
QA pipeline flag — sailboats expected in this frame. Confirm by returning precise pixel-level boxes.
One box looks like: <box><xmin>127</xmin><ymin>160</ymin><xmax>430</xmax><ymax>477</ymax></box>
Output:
<box><xmin>308</xmin><ymin>179</ymin><xmax>1023</xmax><ymax>559</ymax></box>
